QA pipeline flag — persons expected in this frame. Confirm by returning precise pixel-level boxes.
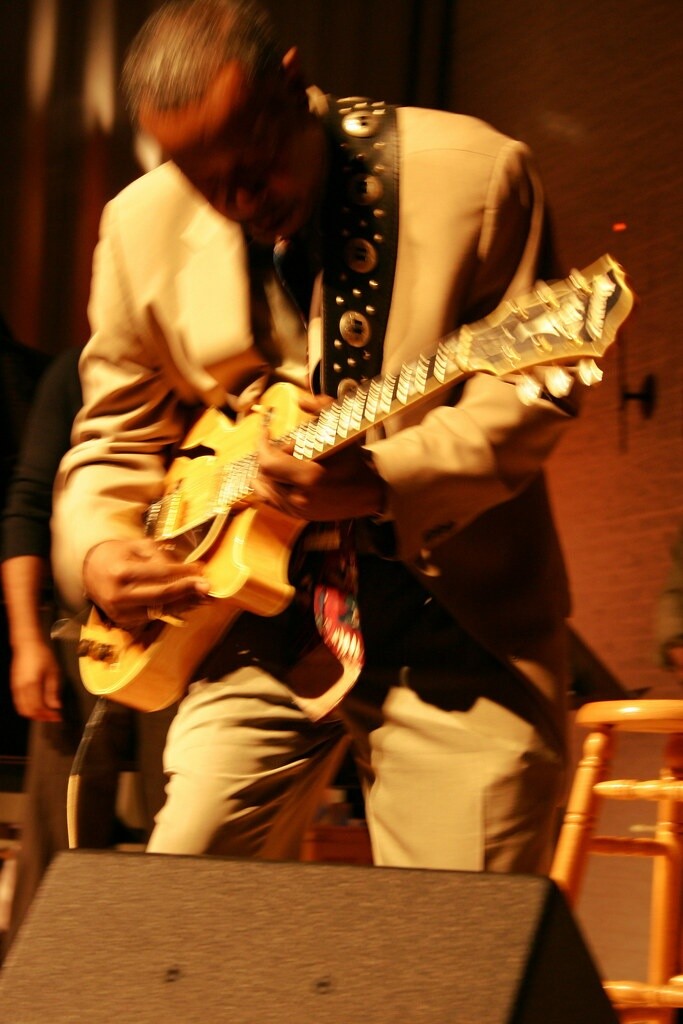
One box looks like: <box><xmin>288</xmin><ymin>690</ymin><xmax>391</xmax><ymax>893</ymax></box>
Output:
<box><xmin>50</xmin><ymin>0</ymin><xmax>574</xmax><ymax>876</ymax></box>
<box><xmin>0</xmin><ymin>352</ymin><xmax>182</xmax><ymax>949</ymax></box>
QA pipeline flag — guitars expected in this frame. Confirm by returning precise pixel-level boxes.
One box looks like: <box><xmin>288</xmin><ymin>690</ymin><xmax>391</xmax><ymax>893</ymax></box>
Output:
<box><xmin>72</xmin><ymin>247</ymin><xmax>640</xmax><ymax>715</ymax></box>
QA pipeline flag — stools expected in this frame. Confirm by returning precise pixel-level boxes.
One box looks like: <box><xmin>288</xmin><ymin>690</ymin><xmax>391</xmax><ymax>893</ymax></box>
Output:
<box><xmin>550</xmin><ymin>699</ymin><xmax>683</xmax><ymax>1010</ymax></box>
